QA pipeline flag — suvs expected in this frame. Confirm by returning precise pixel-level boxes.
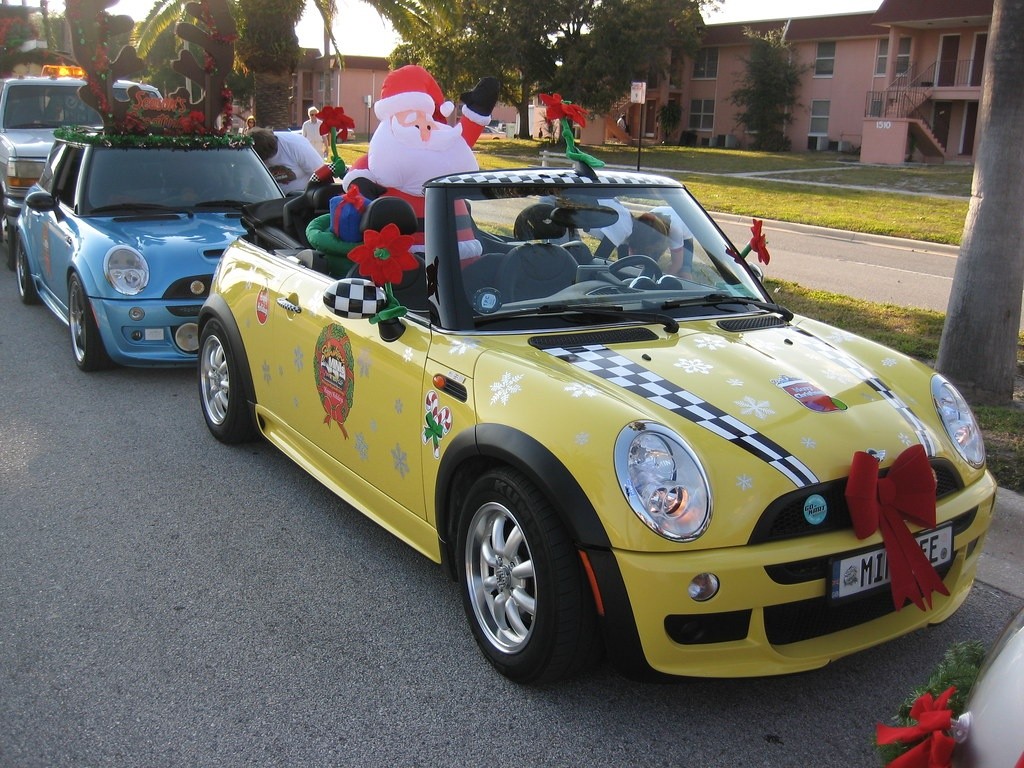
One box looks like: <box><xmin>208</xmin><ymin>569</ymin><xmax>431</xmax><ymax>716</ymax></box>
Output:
<box><xmin>1</xmin><ymin>65</ymin><xmax>169</xmax><ymax>273</ymax></box>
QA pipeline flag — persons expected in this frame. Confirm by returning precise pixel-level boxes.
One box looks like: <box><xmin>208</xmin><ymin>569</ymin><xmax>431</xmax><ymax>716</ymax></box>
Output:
<box><xmin>539</xmin><ymin>185</ymin><xmax>695</xmax><ymax>282</ymax></box>
<box><xmin>301</xmin><ymin>106</ymin><xmax>330</xmax><ymax>161</ymax></box>
<box><xmin>244</xmin><ymin>116</ymin><xmax>256</xmax><ymax>136</ymax></box>
<box><xmin>616</xmin><ymin>114</ymin><xmax>627</xmax><ymax>133</ymax></box>
<box><xmin>245</xmin><ymin>126</ymin><xmax>325</xmax><ymax>198</ymax></box>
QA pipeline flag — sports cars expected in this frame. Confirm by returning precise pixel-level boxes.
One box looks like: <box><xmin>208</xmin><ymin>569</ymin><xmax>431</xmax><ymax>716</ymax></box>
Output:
<box><xmin>194</xmin><ymin>167</ymin><xmax>999</xmax><ymax>688</ymax></box>
<box><xmin>13</xmin><ymin>125</ymin><xmax>292</xmax><ymax>374</ymax></box>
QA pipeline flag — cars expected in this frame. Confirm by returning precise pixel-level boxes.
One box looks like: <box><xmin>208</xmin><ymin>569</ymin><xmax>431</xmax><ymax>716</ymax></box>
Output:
<box><xmin>336</xmin><ymin>129</ymin><xmax>357</xmax><ymax>145</ymax></box>
<box><xmin>476</xmin><ymin>125</ymin><xmax>509</xmax><ymax>140</ymax></box>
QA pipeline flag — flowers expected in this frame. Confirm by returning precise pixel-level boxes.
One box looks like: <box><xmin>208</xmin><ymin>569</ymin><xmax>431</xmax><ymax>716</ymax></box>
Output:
<box><xmin>316</xmin><ymin>102</ymin><xmax>355</xmax><ymax>177</ymax></box>
<box><xmin>538</xmin><ymin>92</ymin><xmax>607</xmax><ymax>167</ymax></box>
<box><xmin>739</xmin><ymin>220</ymin><xmax>771</xmax><ymax>265</ymax></box>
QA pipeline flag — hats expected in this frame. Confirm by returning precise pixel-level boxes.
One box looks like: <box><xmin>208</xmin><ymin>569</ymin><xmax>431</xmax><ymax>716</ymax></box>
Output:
<box><xmin>373</xmin><ymin>65</ymin><xmax>455</xmax><ymax>121</ymax></box>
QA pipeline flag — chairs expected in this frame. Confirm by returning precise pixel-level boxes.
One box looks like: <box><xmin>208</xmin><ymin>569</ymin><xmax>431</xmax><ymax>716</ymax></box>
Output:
<box><xmin>496</xmin><ymin>242</ymin><xmax>578</xmax><ymax>304</ymax></box>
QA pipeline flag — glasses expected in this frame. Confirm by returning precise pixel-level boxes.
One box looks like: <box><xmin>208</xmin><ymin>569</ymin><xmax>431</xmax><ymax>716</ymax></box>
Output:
<box><xmin>249</xmin><ymin>122</ymin><xmax>254</xmax><ymax>123</ymax></box>
<box><xmin>310</xmin><ymin>114</ymin><xmax>317</xmax><ymax>116</ymax></box>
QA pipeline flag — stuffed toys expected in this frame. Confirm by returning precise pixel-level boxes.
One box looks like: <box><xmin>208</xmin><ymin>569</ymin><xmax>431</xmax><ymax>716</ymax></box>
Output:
<box><xmin>343</xmin><ymin>64</ymin><xmax>501</xmax><ymax>270</ymax></box>
